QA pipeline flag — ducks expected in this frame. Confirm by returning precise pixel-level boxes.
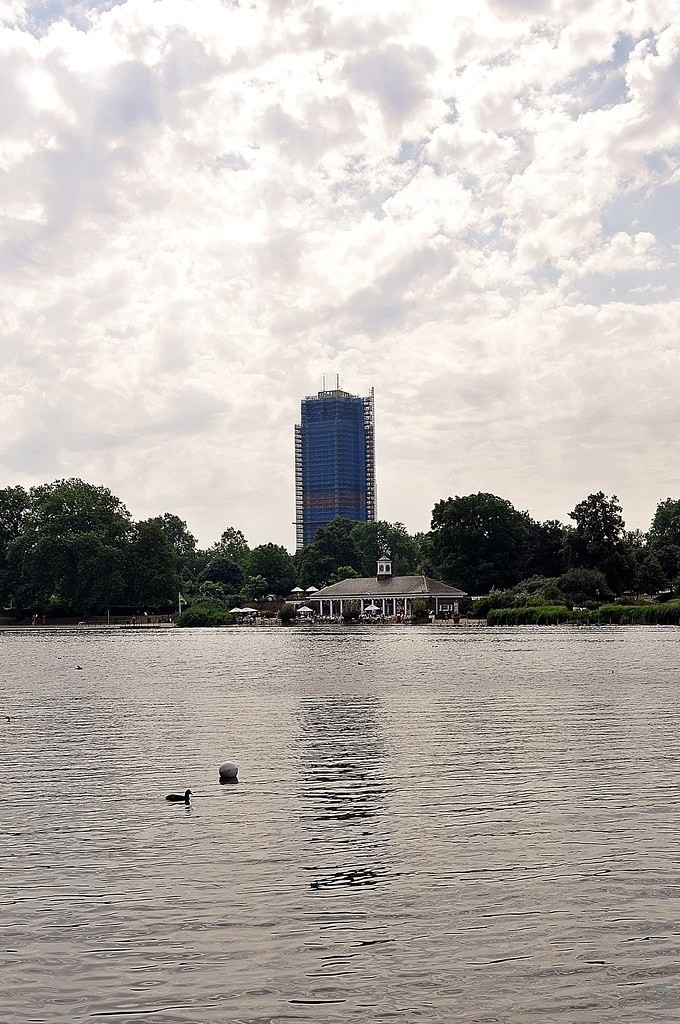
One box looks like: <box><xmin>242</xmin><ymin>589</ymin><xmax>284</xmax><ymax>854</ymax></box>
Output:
<box><xmin>165</xmin><ymin>789</ymin><xmax>194</xmax><ymax>801</ymax></box>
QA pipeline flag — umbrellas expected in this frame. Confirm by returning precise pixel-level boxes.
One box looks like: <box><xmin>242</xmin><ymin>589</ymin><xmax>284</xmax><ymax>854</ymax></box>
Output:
<box><xmin>365</xmin><ymin>604</ymin><xmax>380</xmax><ymax>613</ymax></box>
<box><xmin>306</xmin><ymin>586</ymin><xmax>319</xmax><ymax>592</ymax></box>
<box><xmin>297</xmin><ymin>606</ymin><xmax>314</xmax><ymax>616</ymax></box>
<box><xmin>291</xmin><ymin>587</ymin><xmax>304</xmax><ymax>600</ymax></box>
<box><xmin>229</xmin><ymin>607</ymin><xmax>242</xmax><ymax>616</ymax></box>
<box><xmin>241</xmin><ymin>607</ymin><xmax>257</xmax><ymax>614</ymax></box>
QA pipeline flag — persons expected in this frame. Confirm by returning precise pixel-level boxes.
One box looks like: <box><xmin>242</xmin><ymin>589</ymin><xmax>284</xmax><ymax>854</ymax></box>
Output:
<box><xmin>234</xmin><ymin>610</ymin><xmax>406</xmax><ymax>625</ymax></box>
<box><xmin>131</xmin><ymin>614</ymin><xmax>137</xmax><ymax>625</ymax></box>
<box><xmin>427</xmin><ymin>609</ymin><xmax>472</xmax><ymax>624</ymax></box>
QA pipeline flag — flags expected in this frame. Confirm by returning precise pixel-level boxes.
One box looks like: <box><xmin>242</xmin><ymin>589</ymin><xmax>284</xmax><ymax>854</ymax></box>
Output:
<box><xmin>179</xmin><ymin>594</ymin><xmax>187</xmax><ymax>604</ymax></box>
